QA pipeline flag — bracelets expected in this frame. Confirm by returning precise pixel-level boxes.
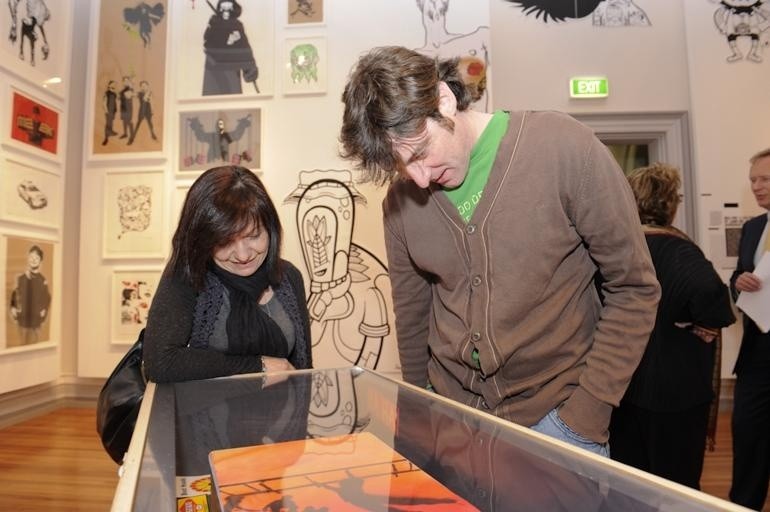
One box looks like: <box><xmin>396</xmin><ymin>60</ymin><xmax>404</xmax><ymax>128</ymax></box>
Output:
<box><xmin>261</xmin><ymin>356</ymin><xmax>266</xmax><ymax>372</ymax></box>
<box><xmin>261</xmin><ymin>375</ymin><xmax>267</xmax><ymax>390</ymax></box>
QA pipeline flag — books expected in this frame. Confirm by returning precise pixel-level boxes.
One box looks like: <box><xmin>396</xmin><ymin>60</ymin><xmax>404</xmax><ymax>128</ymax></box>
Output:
<box><xmin>734</xmin><ymin>251</ymin><xmax>770</xmax><ymax>335</ymax></box>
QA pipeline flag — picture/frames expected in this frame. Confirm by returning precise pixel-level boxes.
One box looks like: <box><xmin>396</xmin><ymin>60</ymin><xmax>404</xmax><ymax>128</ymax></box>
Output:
<box><xmin>108</xmin><ymin>268</ymin><xmax>163</xmax><ymax>345</ymax></box>
<box><xmin>284</xmin><ymin>0</ymin><xmax>328</xmax><ymax>27</ymax></box>
<box><xmin>84</xmin><ymin>0</ymin><xmax>172</xmax><ymax>168</ymax></box>
<box><xmin>103</xmin><ymin>167</ymin><xmax>170</xmax><ymax>261</ymax></box>
<box><xmin>283</xmin><ymin>35</ymin><xmax>328</xmax><ymax>95</ymax></box>
<box><xmin>175</xmin><ymin>2</ymin><xmax>276</xmax><ymax>103</ymax></box>
<box><xmin>0</xmin><ymin>0</ymin><xmax>69</xmax><ymax>101</ymax></box>
<box><xmin>2</xmin><ymin>154</ymin><xmax>64</xmax><ymax>232</ymax></box>
<box><xmin>0</xmin><ymin>232</ymin><xmax>63</xmax><ymax>358</ymax></box>
<box><xmin>174</xmin><ymin>106</ymin><xmax>266</xmax><ymax>178</ymax></box>
<box><xmin>0</xmin><ymin>81</ymin><xmax>65</xmax><ymax>166</ymax></box>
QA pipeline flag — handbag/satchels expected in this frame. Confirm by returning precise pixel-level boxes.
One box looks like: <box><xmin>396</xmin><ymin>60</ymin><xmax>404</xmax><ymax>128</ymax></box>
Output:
<box><xmin>96</xmin><ymin>340</ymin><xmax>147</xmax><ymax>466</ymax></box>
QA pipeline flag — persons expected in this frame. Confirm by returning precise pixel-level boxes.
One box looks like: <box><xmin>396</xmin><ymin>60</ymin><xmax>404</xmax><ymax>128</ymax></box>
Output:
<box><xmin>102</xmin><ymin>81</ymin><xmax>119</xmax><ymax>145</ymax></box>
<box><xmin>189</xmin><ymin>116</ymin><xmax>251</xmax><ymax>163</ymax></box>
<box><xmin>202</xmin><ymin>1</ymin><xmax>260</xmax><ymax>97</ymax></box>
<box><xmin>9</xmin><ymin>245</ymin><xmax>52</xmax><ymax>345</ymax></box>
<box><xmin>386</xmin><ymin>386</ymin><xmax>607</xmax><ymax>511</ymax></box>
<box><xmin>727</xmin><ymin>149</ymin><xmax>769</xmax><ymax>512</ymax></box>
<box><xmin>139</xmin><ymin>164</ymin><xmax>315</xmax><ymax>477</ymax></box>
<box><xmin>334</xmin><ymin>44</ymin><xmax>667</xmax><ymax>461</ymax></box>
<box><xmin>593</xmin><ymin>158</ymin><xmax>736</xmax><ymax>492</ymax></box>
<box><xmin>147</xmin><ymin>371</ymin><xmax>312</xmax><ymax>505</ymax></box>
<box><xmin>118</xmin><ymin>77</ymin><xmax>134</xmax><ymax>139</ymax></box>
<box><xmin>127</xmin><ymin>81</ymin><xmax>157</xmax><ymax>145</ymax></box>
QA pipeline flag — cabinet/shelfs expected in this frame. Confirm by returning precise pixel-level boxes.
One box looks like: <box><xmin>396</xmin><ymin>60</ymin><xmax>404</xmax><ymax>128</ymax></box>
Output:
<box><xmin>112</xmin><ymin>363</ymin><xmax>762</xmax><ymax>512</ymax></box>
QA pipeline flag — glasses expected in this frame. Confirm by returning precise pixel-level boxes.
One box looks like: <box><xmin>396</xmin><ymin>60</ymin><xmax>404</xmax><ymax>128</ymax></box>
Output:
<box><xmin>672</xmin><ymin>192</ymin><xmax>684</xmax><ymax>202</ymax></box>
